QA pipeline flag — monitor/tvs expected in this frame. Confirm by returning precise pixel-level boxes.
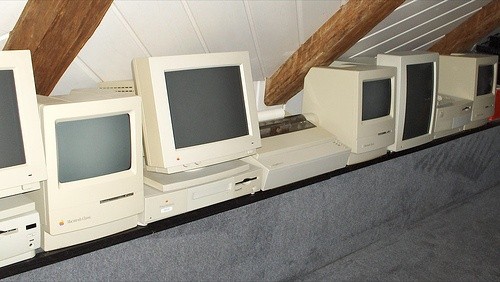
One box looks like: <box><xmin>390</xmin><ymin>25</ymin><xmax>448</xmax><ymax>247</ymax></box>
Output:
<box><xmin>0</xmin><ymin>49</ymin><xmax>499</xmax><ymax>268</ymax></box>
